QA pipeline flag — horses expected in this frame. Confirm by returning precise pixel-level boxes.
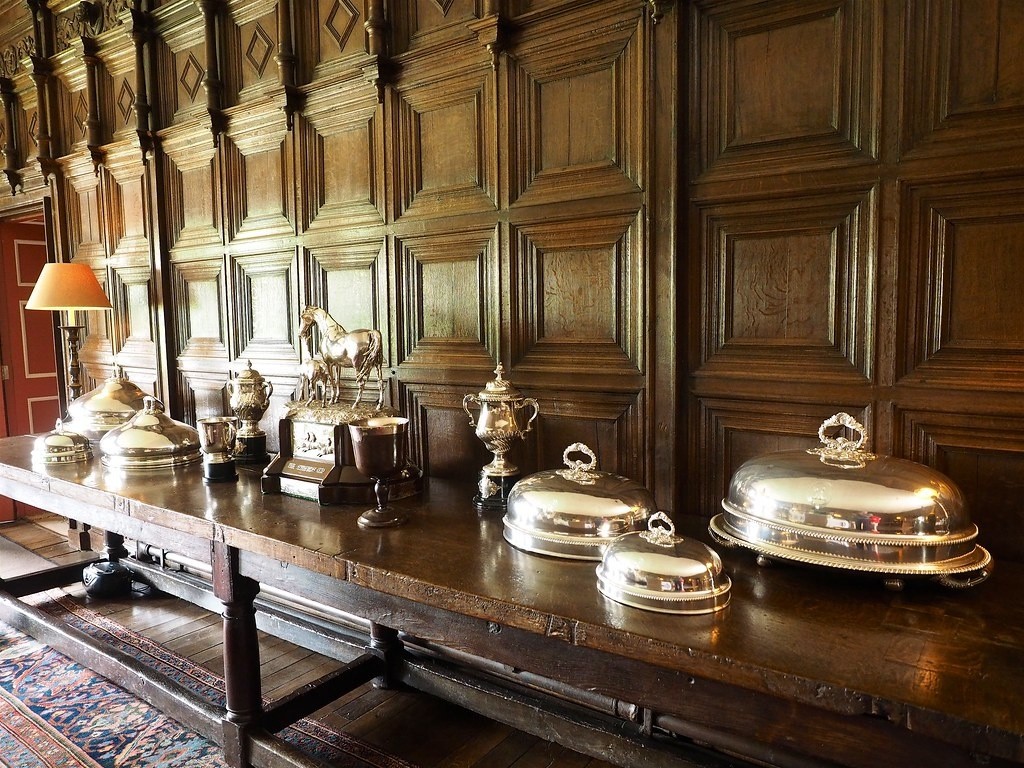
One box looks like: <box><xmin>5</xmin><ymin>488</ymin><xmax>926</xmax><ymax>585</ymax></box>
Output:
<box><xmin>296</xmin><ymin>303</ymin><xmax>385</xmax><ymax>413</ymax></box>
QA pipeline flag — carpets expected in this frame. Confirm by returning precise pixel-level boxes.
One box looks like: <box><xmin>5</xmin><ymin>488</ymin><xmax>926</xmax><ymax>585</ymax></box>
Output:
<box><xmin>0</xmin><ymin>535</ymin><xmax>416</xmax><ymax>768</ymax></box>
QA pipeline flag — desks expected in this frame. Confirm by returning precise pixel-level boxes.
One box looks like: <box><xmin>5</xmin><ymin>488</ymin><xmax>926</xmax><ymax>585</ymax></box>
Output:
<box><xmin>0</xmin><ymin>430</ymin><xmax>1024</xmax><ymax>768</ymax></box>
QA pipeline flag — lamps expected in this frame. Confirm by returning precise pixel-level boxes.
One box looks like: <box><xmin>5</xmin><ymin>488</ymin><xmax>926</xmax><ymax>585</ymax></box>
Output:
<box><xmin>24</xmin><ymin>263</ymin><xmax>113</xmax><ymax>425</ymax></box>
